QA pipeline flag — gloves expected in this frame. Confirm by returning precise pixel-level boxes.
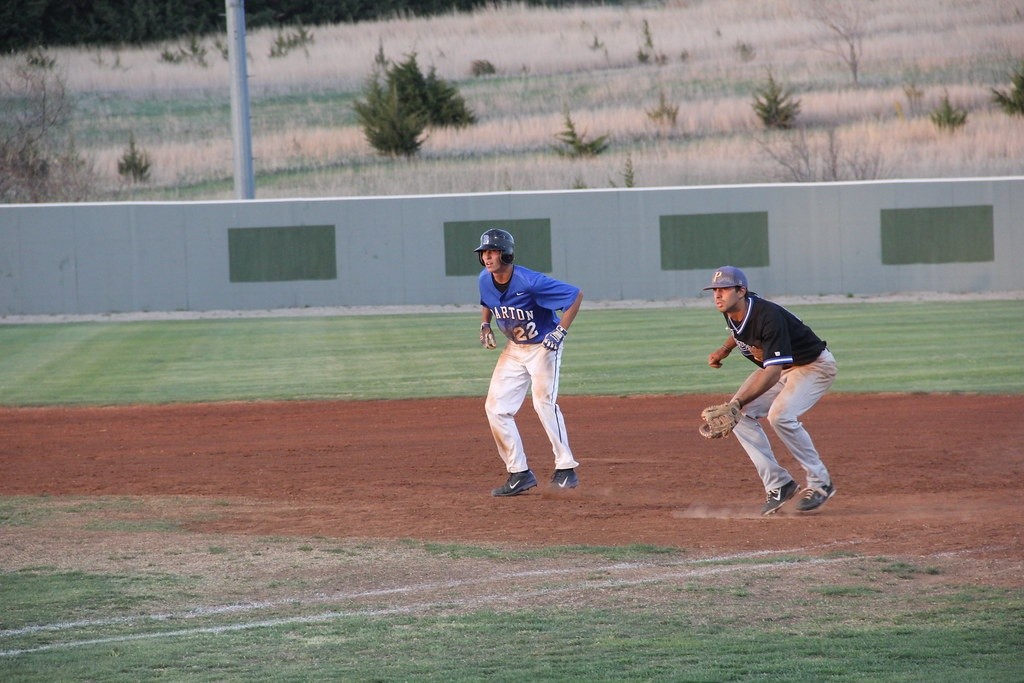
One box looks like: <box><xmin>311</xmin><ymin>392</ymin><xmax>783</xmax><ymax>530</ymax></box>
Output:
<box><xmin>480</xmin><ymin>322</ymin><xmax>496</xmax><ymax>349</ymax></box>
<box><xmin>542</xmin><ymin>324</ymin><xmax>567</xmax><ymax>351</ymax></box>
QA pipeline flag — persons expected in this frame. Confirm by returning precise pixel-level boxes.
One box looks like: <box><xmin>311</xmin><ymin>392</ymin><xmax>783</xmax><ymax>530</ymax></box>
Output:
<box><xmin>699</xmin><ymin>266</ymin><xmax>837</xmax><ymax>516</ymax></box>
<box><xmin>474</xmin><ymin>228</ymin><xmax>583</xmax><ymax>496</ymax></box>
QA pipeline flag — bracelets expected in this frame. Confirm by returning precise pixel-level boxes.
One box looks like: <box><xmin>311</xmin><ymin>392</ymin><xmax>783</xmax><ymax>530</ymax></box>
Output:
<box><xmin>722</xmin><ymin>345</ymin><xmax>731</xmax><ymax>352</ymax></box>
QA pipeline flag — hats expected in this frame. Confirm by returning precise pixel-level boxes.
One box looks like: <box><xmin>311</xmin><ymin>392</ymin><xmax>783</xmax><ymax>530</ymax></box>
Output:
<box><xmin>703</xmin><ymin>266</ymin><xmax>748</xmax><ymax>290</ymax></box>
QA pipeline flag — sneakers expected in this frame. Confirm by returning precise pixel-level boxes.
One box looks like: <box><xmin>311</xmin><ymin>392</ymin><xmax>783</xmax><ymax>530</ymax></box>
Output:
<box><xmin>491</xmin><ymin>471</ymin><xmax>538</xmax><ymax>496</ymax></box>
<box><xmin>794</xmin><ymin>478</ymin><xmax>836</xmax><ymax>513</ymax></box>
<box><xmin>761</xmin><ymin>479</ymin><xmax>800</xmax><ymax>516</ymax></box>
<box><xmin>549</xmin><ymin>468</ymin><xmax>578</xmax><ymax>489</ymax></box>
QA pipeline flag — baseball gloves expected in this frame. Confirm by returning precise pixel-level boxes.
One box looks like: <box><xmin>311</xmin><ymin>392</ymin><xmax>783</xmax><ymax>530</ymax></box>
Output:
<box><xmin>698</xmin><ymin>401</ymin><xmax>742</xmax><ymax>440</ymax></box>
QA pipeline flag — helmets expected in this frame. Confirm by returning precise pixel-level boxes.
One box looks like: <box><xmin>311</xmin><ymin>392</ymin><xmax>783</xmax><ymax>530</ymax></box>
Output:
<box><xmin>473</xmin><ymin>228</ymin><xmax>515</xmax><ymax>266</ymax></box>
